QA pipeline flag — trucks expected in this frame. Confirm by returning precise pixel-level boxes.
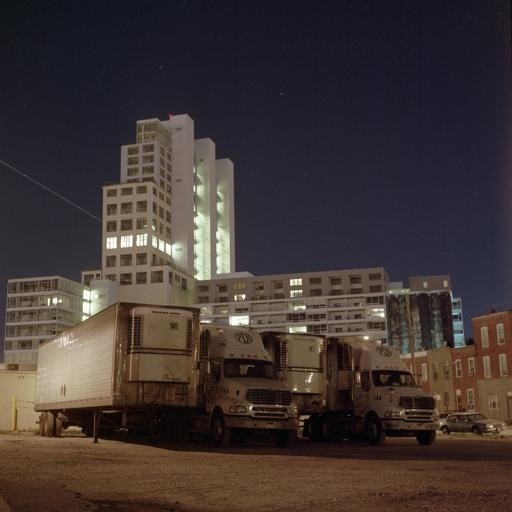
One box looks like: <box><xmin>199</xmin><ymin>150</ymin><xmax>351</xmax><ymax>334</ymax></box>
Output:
<box><xmin>259</xmin><ymin>330</ymin><xmax>440</xmax><ymax>446</ymax></box>
<box><xmin>34</xmin><ymin>302</ymin><xmax>299</xmax><ymax>450</ymax></box>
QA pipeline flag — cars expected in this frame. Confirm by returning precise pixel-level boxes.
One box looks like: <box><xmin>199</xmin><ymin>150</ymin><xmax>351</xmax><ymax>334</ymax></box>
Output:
<box><xmin>437</xmin><ymin>412</ymin><xmax>506</xmax><ymax>435</ymax></box>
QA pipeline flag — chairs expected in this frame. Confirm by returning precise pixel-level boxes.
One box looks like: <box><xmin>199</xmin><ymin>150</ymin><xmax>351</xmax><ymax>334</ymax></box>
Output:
<box><xmin>374</xmin><ymin>374</ymin><xmax>403</xmax><ymax>385</ymax></box>
<box><xmin>228</xmin><ymin>365</ymin><xmax>263</xmax><ymax>377</ymax></box>
